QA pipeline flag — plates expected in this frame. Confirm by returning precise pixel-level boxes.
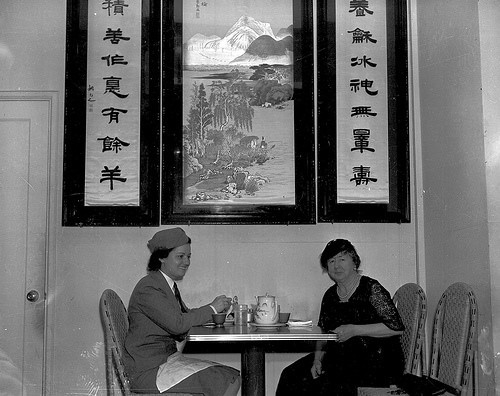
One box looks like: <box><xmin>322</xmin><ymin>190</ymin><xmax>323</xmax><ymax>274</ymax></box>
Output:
<box><xmin>249</xmin><ymin>322</ymin><xmax>286</xmax><ymax>327</ymax></box>
<box><xmin>287</xmin><ymin>319</ymin><xmax>312</xmax><ymax>326</ymax></box>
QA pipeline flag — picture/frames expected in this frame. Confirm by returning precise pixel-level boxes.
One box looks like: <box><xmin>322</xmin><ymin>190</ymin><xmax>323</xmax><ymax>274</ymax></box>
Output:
<box><xmin>62</xmin><ymin>0</ymin><xmax>160</xmax><ymax>228</ymax></box>
<box><xmin>315</xmin><ymin>0</ymin><xmax>412</xmax><ymax>225</ymax></box>
<box><xmin>160</xmin><ymin>0</ymin><xmax>317</xmax><ymax>226</ymax></box>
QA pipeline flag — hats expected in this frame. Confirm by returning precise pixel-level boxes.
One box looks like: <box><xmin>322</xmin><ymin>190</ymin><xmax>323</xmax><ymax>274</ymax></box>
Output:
<box><xmin>146</xmin><ymin>227</ymin><xmax>192</xmax><ymax>253</ymax></box>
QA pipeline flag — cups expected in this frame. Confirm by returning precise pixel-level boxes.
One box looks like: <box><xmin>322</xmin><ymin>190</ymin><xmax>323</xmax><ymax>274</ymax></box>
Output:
<box><xmin>279</xmin><ymin>313</ymin><xmax>291</xmax><ymax>323</ymax></box>
<box><xmin>233</xmin><ymin>304</ymin><xmax>247</xmax><ymax>326</ymax></box>
<box><xmin>212</xmin><ymin>313</ymin><xmax>226</xmax><ymax>328</ymax></box>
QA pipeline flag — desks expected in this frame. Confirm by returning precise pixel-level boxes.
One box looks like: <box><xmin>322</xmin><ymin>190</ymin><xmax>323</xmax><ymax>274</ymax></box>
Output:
<box><xmin>182</xmin><ymin>323</ymin><xmax>338</xmax><ymax>396</ymax></box>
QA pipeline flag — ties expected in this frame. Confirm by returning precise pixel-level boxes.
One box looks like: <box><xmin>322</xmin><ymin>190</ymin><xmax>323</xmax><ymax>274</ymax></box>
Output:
<box><xmin>173</xmin><ymin>281</ymin><xmax>187</xmax><ymax>313</ymax></box>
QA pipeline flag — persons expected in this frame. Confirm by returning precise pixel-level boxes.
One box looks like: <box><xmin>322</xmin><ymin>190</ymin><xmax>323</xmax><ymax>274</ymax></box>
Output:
<box><xmin>121</xmin><ymin>227</ymin><xmax>240</xmax><ymax>396</ymax></box>
<box><xmin>276</xmin><ymin>238</ymin><xmax>406</xmax><ymax>396</ymax></box>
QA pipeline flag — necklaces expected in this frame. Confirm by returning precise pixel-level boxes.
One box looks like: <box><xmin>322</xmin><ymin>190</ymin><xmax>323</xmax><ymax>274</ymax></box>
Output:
<box><xmin>337</xmin><ymin>275</ymin><xmax>359</xmax><ymax>299</ymax></box>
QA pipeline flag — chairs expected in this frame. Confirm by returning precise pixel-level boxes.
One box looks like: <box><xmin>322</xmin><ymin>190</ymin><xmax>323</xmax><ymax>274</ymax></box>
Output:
<box><xmin>98</xmin><ymin>289</ymin><xmax>202</xmax><ymax>396</ymax></box>
<box><xmin>357</xmin><ymin>283</ymin><xmax>480</xmax><ymax>396</ymax></box>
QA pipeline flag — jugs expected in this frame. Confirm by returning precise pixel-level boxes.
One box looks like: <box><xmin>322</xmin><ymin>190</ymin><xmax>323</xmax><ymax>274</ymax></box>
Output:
<box><xmin>254</xmin><ymin>294</ymin><xmax>280</xmax><ymax>324</ymax></box>
